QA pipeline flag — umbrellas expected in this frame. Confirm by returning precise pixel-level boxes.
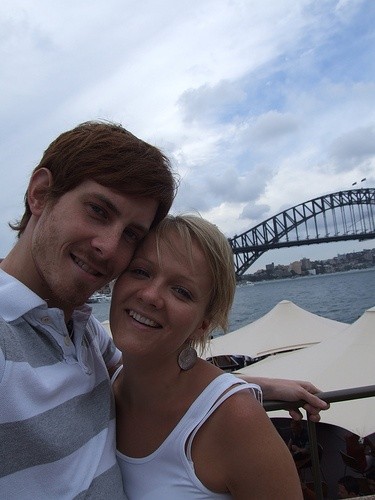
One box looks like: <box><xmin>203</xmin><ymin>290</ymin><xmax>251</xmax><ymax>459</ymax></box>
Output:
<box><xmin>193</xmin><ymin>300</ymin><xmax>375</xmax><ymax>437</ymax></box>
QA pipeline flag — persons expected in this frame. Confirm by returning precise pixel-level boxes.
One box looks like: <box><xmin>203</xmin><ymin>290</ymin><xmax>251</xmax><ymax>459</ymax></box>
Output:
<box><xmin>288</xmin><ymin>418</ymin><xmax>375</xmax><ymax>498</ymax></box>
<box><xmin>0</xmin><ymin>121</ymin><xmax>331</xmax><ymax>500</ymax></box>
<box><xmin>109</xmin><ymin>213</ymin><xmax>304</xmax><ymax>500</ymax></box>
